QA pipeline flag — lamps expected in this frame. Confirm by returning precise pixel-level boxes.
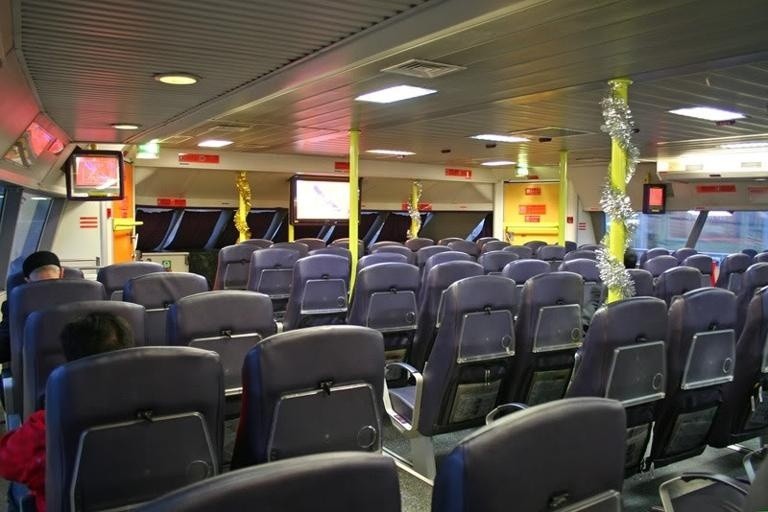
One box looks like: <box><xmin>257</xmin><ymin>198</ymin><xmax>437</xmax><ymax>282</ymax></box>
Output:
<box><xmin>154</xmin><ymin>71</ymin><xmax>199</xmax><ymax>87</ymax></box>
<box><xmin>108</xmin><ymin>121</ymin><xmax>142</xmax><ymax>132</ymax></box>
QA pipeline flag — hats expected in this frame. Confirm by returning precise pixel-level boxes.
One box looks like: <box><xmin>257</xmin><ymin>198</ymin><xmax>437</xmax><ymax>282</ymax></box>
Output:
<box><xmin>22</xmin><ymin>251</ymin><xmax>61</xmax><ymax>278</ymax></box>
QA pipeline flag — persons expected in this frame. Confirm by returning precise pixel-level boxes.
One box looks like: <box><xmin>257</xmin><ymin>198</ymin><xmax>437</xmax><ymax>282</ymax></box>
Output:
<box><xmin>0</xmin><ymin>310</ymin><xmax>133</xmax><ymax>512</ymax></box>
<box><xmin>0</xmin><ymin>250</ymin><xmax>64</xmax><ymax>364</ymax></box>
<box><xmin>624</xmin><ymin>248</ymin><xmax>637</xmax><ymax>269</ymax></box>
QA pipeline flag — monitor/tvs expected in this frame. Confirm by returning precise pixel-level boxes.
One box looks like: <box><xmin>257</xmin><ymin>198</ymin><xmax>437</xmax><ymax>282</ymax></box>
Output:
<box><xmin>5</xmin><ymin>123</ymin><xmax>57</xmax><ymax>172</ymax></box>
<box><xmin>290</xmin><ymin>173</ymin><xmax>364</xmax><ymax>227</ymax></box>
<box><xmin>66</xmin><ymin>148</ymin><xmax>124</xmax><ymax>202</ymax></box>
<box><xmin>643</xmin><ymin>183</ymin><xmax>667</xmax><ymax>216</ymax></box>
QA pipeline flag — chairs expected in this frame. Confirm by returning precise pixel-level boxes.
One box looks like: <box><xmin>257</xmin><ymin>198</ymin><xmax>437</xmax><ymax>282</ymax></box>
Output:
<box><xmin>308</xmin><ymin>247</ymin><xmax>353</xmax><ymax>280</ymax></box>
<box><xmin>46</xmin><ymin>346</ymin><xmax>225</xmax><ymax>512</ymax></box>
<box><xmin>557</xmin><ymin>258</ymin><xmax>601</xmax><ymax>305</ymax></box>
<box><xmin>420</xmin><ymin>251</ymin><xmax>471</xmax><ymax>298</ymax></box>
<box><xmin>753</xmin><ymin>253</ymin><xmax>768</xmax><ymax>263</ymax></box>
<box><xmin>245</xmin><ymin>247</ymin><xmax>301</xmax><ymax>319</ymax></box>
<box><xmin>502</xmin><ymin>258</ymin><xmax>551</xmax><ymax>292</ymax></box>
<box><xmin>476</xmin><ymin>237</ymin><xmax>500</xmax><ymax>252</ymax></box>
<box><xmin>270</xmin><ymin>242</ymin><xmax>308</xmax><ymax>258</ymax></box>
<box><xmin>715</xmin><ymin>253</ymin><xmax>752</xmax><ymax>295</ymax></box>
<box><xmin>502</xmin><ymin>245</ymin><xmax>533</xmax><ymax>260</ymax></box>
<box><xmin>674</xmin><ymin>248</ymin><xmax>697</xmax><ymax>264</ymax></box>
<box><xmin>639</xmin><ymin>254</ymin><xmax>678</xmax><ymax>286</ymax></box>
<box><xmin>372</xmin><ymin>245</ymin><xmax>416</xmax><ymax>264</ymax></box>
<box><xmin>124</xmin><ymin>271</ymin><xmax>209</xmax><ymax>345</ymax></box>
<box><xmin>416</xmin><ymin>244</ymin><xmax>452</xmax><ymax>266</ymax></box>
<box><xmin>599</xmin><ymin>268</ymin><xmax>654</xmax><ymax>305</ymax></box>
<box><xmin>482</xmin><ymin>295</ymin><xmax>668</xmax><ymax>478</ymax></box>
<box><xmin>707</xmin><ymin>286</ymin><xmax>767</xmax><ymax>448</ymax></box>
<box><xmin>165</xmin><ymin>288</ymin><xmax>273</xmax><ymax>418</ymax></box>
<box><xmin>537</xmin><ymin>245</ymin><xmax>565</xmax><ymax>272</ymax></box>
<box><xmin>447</xmin><ymin>241</ymin><xmax>478</xmax><ymax>260</ymax></box>
<box><xmin>562</xmin><ymin>250</ymin><xmax>596</xmax><ymax>261</ymax></box>
<box><xmin>136</xmin><ymin>450</ymin><xmax>401</xmax><ymax>512</ymax></box>
<box><xmin>477</xmin><ymin>250</ymin><xmax>519</xmax><ymax>275</ymax></box>
<box><xmin>640</xmin><ymin>248</ymin><xmax>670</xmax><ymax>264</ymax></box>
<box><xmin>680</xmin><ymin>254</ymin><xmax>712</xmax><ymax>287</ymax></box>
<box><xmin>239</xmin><ymin>239</ymin><xmax>273</xmax><ymax>249</ymax></box>
<box><xmin>578</xmin><ymin>243</ymin><xmax>598</xmax><ymax>251</ymax></box>
<box><xmin>356</xmin><ymin>253</ymin><xmax>407</xmax><ymax>273</ymax></box>
<box><xmin>655</xmin><ymin>265</ymin><xmax>704</xmax><ymax>308</ymax></box>
<box><xmin>481</xmin><ymin>241</ymin><xmax>510</xmax><ymax>254</ymax></box>
<box><xmin>653</xmin><ymin>285</ymin><xmax>738</xmax><ymax>466</ymax></box>
<box><xmin>405</xmin><ymin>238</ymin><xmax>434</xmax><ymax>252</ymax></box>
<box><xmin>431</xmin><ymin>396</ymin><xmax>626</xmax><ymax>512</ymax></box>
<box><xmin>736</xmin><ymin>261</ymin><xmax>768</xmax><ymax>328</ymax></box>
<box><xmin>328</xmin><ymin>237</ymin><xmax>364</xmax><ymax>257</ymax></box>
<box><xmin>411</xmin><ymin>260</ymin><xmax>484</xmax><ymax>370</ymax></box>
<box><xmin>296</xmin><ymin>238</ymin><xmax>326</xmax><ymax>251</ymax></box>
<box><xmin>523</xmin><ymin>241</ymin><xmax>547</xmax><ymax>256</ymax></box>
<box><xmin>649</xmin><ymin>447</ymin><xmax>768</xmax><ymax>512</ymax></box>
<box><xmin>504</xmin><ymin>271</ymin><xmax>585</xmax><ymax>408</ymax></box>
<box><xmin>348</xmin><ymin>262</ymin><xmax>420</xmax><ymax>381</ymax></box>
<box><xmin>739</xmin><ymin>249</ymin><xmax>757</xmax><ymax>257</ymax></box>
<box><xmin>230</xmin><ymin>324</ymin><xmax>385</xmax><ymax>469</ymax></box>
<box><xmin>7</xmin><ymin>266</ymin><xmax>84</xmax><ymax>298</ymax></box>
<box><xmin>438</xmin><ymin>237</ymin><xmax>463</xmax><ymax>246</ymax></box>
<box><xmin>98</xmin><ymin>261</ymin><xmax>161</xmax><ymax>303</ymax></box>
<box><xmin>23</xmin><ymin>300</ymin><xmax>146</xmax><ymax>429</ymax></box>
<box><xmin>371</xmin><ymin>241</ymin><xmax>402</xmax><ymax>250</ymax></box>
<box><xmin>211</xmin><ymin>244</ymin><xmax>262</xmax><ymax>291</ymax></box>
<box><xmin>275</xmin><ymin>253</ymin><xmax>349</xmax><ymax>335</ymax></box>
<box><xmin>381</xmin><ymin>274</ymin><xmax>517</xmax><ymax>486</ymax></box>
<box><xmin>2</xmin><ymin>279</ymin><xmax>108</xmax><ymax>413</ymax></box>
